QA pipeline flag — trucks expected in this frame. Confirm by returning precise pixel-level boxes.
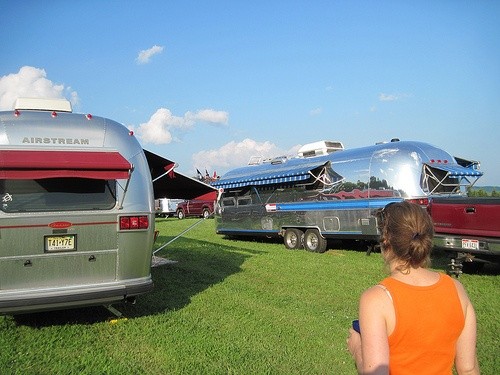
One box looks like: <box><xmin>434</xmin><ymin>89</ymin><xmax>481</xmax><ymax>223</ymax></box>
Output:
<box><xmin>0</xmin><ymin>97</ymin><xmax>158</xmax><ymax>318</ymax></box>
<box><xmin>429</xmin><ymin>193</ymin><xmax>500</xmax><ymax>257</ymax></box>
<box><xmin>154</xmin><ymin>199</ymin><xmax>184</xmax><ymax>218</ymax></box>
<box><xmin>210</xmin><ymin>137</ymin><xmax>484</xmax><ymax>253</ymax></box>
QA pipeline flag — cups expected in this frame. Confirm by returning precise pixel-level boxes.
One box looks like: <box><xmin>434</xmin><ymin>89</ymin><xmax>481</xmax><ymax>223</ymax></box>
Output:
<box><xmin>352</xmin><ymin>320</ymin><xmax>361</xmax><ymax>335</ymax></box>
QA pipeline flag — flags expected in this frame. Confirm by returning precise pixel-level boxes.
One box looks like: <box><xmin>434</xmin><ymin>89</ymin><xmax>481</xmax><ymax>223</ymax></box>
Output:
<box><xmin>196</xmin><ymin>167</ymin><xmax>217</xmax><ymax>183</ymax></box>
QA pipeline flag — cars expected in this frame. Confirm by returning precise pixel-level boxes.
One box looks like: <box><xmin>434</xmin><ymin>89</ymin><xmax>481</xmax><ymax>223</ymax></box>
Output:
<box><xmin>175</xmin><ymin>199</ymin><xmax>215</xmax><ymax>219</ymax></box>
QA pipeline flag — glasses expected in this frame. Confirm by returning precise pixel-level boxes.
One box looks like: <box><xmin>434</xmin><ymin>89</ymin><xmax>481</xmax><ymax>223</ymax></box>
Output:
<box><xmin>377</xmin><ymin>201</ymin><xmax>399</xmax><ymax>230</ymax></box>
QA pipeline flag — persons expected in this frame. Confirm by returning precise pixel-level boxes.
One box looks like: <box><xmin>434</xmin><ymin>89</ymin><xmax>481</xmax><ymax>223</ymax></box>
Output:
<box><xmin>346</xmin><ymin>201</ymin><xmax>481</xmax><ymax>375</ymax></box>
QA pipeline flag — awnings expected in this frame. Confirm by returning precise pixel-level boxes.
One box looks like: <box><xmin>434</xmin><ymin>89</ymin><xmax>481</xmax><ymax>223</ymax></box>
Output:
<box><xmin>211</xmin><ymin>159</ymin><xmax>331</xmax><ymax>195</ymax></box>
<box><xmin>425</xmin><ymin>154</ymin><xmax>484</xmax><ymax>196</ymax></box>
<box><xmin>0</xmin><ymin>149</ymin><xmax>134</xmax><ymax>209</ymax></box>
<box><xmin>326</xmin><ymin>167</ymin><xmax>345</xmax><ymax>191</ymax></box>
